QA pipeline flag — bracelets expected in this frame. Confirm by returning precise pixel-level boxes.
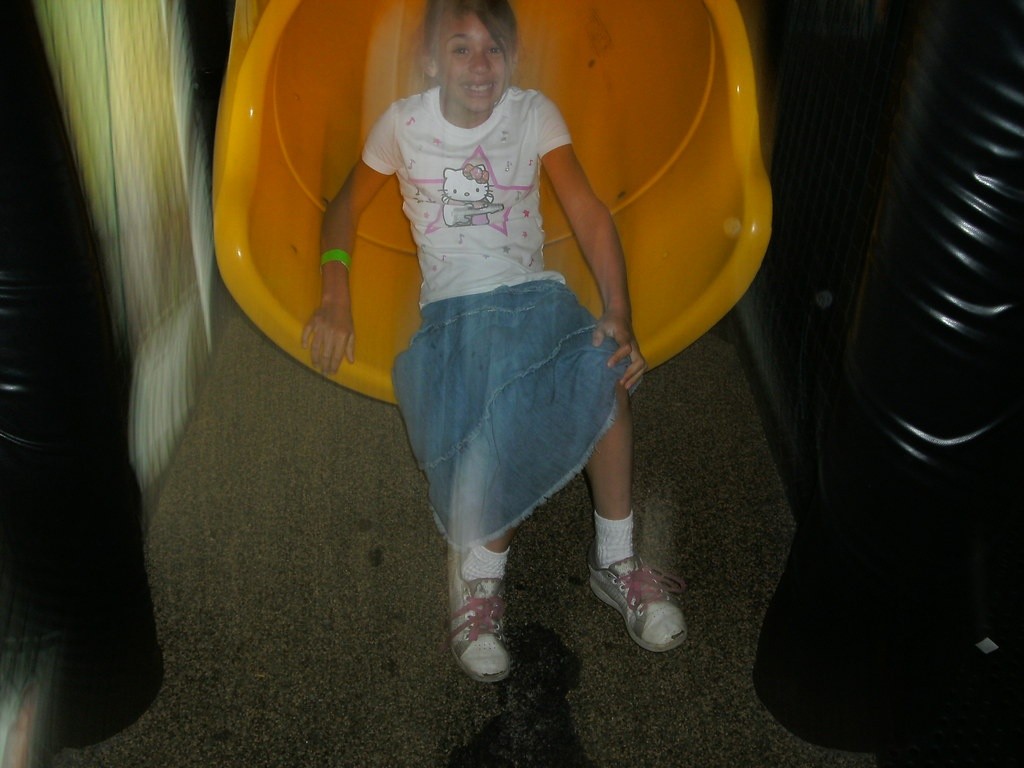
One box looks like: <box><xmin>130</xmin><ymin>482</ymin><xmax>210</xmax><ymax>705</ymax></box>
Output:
<box><xmin>319</xmin><ymin>249</ymin><xmax>352</xmax><ymax>275</ymax></box>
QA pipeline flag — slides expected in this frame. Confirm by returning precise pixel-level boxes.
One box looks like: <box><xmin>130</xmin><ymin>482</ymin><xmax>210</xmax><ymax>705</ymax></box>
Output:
<box><xmin>212</xmin><ymin>3</ymin><xmax>774</xmax><ymax>405</ymax></box>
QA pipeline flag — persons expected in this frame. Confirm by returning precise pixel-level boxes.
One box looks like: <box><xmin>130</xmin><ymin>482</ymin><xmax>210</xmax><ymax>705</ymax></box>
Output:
<box><xmin>301</xmin><ymin>1</ymin><xmax>691</xmax><ymax>686</ymax></box>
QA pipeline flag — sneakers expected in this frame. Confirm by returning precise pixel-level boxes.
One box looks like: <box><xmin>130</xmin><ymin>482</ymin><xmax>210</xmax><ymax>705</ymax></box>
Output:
<box><xmin>452</xmin><ymin>565</ymin><xmax>511</xmax><ymax>680</ymax></box>
<box><xmin>587</xmin><ymin>538</ymin><xmax>689</xmax><ymax>650</ymax></box>
